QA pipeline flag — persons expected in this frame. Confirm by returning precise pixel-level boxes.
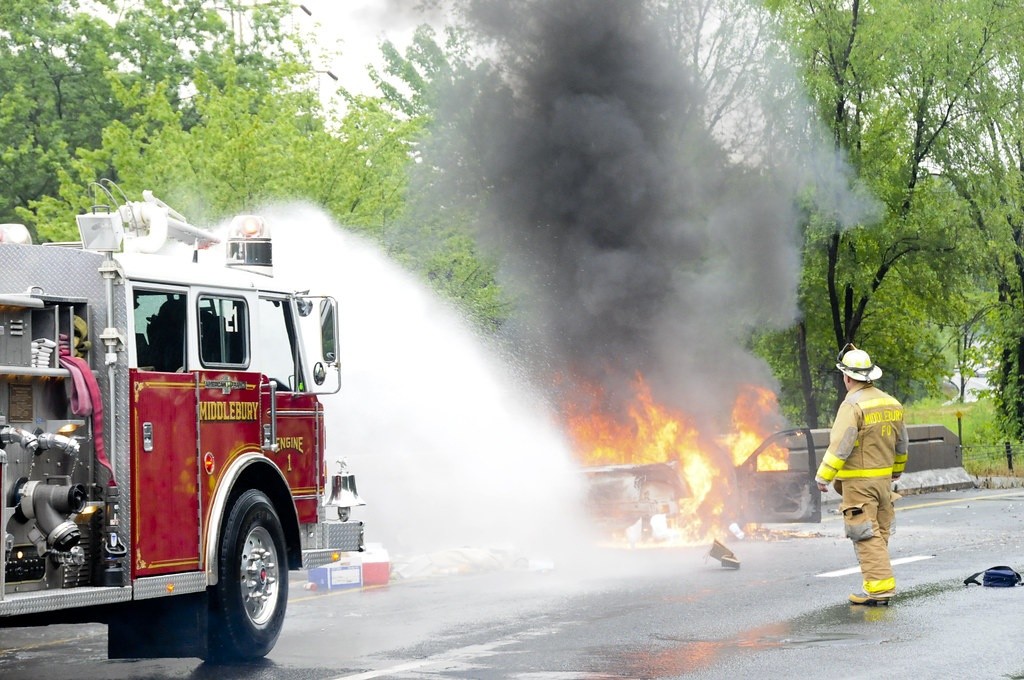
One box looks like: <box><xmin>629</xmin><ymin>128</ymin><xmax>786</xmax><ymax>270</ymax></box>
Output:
<box><xmin>815</xmin><ymin>349</ymin><xmax>910</xmax><ymax>606</ymax></box>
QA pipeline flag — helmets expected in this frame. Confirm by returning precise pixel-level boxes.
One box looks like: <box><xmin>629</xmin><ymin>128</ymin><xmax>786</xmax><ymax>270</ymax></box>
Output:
<box><xmin>835</xmin><ymin>350</ymin><xmax>883</xmax><ymax>381</ymax></box>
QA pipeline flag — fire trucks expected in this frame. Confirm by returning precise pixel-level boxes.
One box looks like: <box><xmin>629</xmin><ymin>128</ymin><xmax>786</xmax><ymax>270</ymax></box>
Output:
<box><xmin>0</xmin><ymin>179</ymin><xmax>363</xmax><ymax>669</ymax></box>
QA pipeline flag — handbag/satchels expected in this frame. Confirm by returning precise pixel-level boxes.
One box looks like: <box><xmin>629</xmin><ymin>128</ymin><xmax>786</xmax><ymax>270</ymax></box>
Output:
<box><xmin>964</xmin><ymin>565</ymin><xmax>1024</xmax><ymax>588</ymax></box>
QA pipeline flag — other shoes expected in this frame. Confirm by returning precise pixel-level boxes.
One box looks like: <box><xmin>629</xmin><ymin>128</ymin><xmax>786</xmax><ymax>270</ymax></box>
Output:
<box><xmin>849</xmin><ymin>592</ymin><xmax>889</xmax><ymax>606</ymax></box>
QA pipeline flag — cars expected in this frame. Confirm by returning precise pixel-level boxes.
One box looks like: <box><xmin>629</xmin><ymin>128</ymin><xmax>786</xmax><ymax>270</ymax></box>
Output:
<box><xmin>570</xmin><ymin>425</ymin><xmax>824</xmax><ymax>541</ymax></box>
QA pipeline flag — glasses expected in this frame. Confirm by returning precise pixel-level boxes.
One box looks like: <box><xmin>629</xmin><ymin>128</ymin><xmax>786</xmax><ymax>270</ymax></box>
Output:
<box><xmin>836</xmin><ymin>343</ymin><xmax>856</xmax><ymax>368</ymax></box>
